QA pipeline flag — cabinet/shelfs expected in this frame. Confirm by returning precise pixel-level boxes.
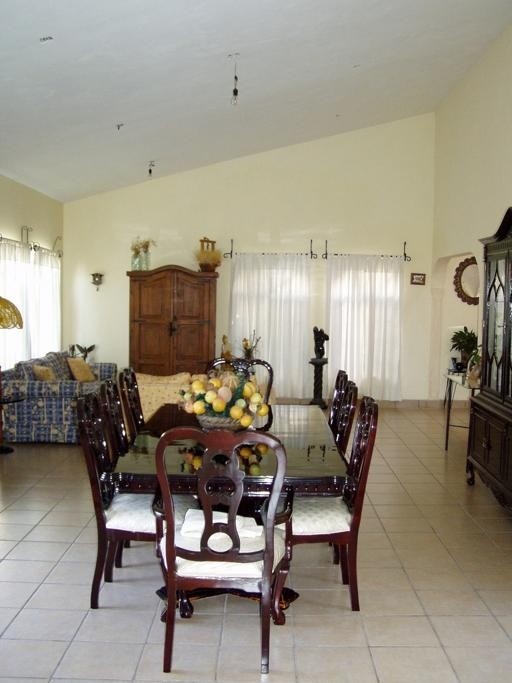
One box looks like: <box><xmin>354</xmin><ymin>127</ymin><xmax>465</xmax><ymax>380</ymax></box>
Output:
<box><xmin>126</xmin><ymin>265</ymin><xmax>220</xmax><ymax>376</ymax></box>
<box><xmin>478</xmin><ymin>205</ymin><xmax>512</xmax><ymax>412</ymax></box>
<box><xmin>464</xmin><ymin>389</ymin><xmax>511</xmax><ymax>512</ymax></box>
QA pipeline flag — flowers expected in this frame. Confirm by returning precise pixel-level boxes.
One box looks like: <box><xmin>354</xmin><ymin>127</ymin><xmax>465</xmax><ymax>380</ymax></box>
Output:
<box><xmin>129</xmin><ymin>239</ymin><xmax>140</xmax><ymax>256</ymax></box>
<box><xmin>140</xmin><ymin>240</ymin><xmax>155</xmax><ymax>250</ymax></box>
<box><xmin>194</xmin><ymin>247</ymin><xmax>224</xmax><ymax>263</ymax></box>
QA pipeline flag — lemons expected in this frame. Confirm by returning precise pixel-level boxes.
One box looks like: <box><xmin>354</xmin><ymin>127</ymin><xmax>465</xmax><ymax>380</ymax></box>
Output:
<box><xmin>184</xmin><ymin>374</ymin><xmax>269</xmax><ymax>427</ymax></box>
<box><xmin>182</xmin><ymin>443</ymin><xmax>269</xmax><ymax>477</ymax></box>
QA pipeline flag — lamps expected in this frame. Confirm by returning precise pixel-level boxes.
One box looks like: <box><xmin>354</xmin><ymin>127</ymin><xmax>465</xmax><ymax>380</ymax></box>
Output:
<box><xmin>226</xmin><ymin>52</ymin><xmax>241</xmax><ymax>105</ymax></box>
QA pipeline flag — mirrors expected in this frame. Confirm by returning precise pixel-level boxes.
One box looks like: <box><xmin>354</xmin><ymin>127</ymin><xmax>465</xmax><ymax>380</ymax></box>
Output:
<box><xmin>452</xmin><ymin>255</ymin><xmax>480</xmax><ymax>304</ymax></box>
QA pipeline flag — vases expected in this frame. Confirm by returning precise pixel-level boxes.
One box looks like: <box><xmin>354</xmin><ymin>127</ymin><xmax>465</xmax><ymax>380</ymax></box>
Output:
<box><xmin>199</xmin><ymin>261</ymin><xmax>218</xmax><ymax>273</ymax></box>
<box><xmin>141</xmin><ymin>251</ymin><xmax>154</xmax><ymax>271</ymax></box>
<box><xmin>130</xmin><ymin>252</ymin><xmax>143</xmax><ymax>271</ymax></box>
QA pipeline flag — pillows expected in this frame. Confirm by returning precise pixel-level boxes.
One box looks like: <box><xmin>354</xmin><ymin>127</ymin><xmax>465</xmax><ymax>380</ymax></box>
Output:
<box><xmin>34</xmin><ymin>365</ymin><xmax>56</xmax><ymax>381</ymax></box>
<box><xmin>67</xmin><ymin>356</ymin><xmax>97</xmax><ymax>381</ymax></box>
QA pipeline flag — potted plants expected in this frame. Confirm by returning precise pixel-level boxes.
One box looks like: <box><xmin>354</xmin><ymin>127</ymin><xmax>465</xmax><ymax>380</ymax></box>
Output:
<box><xmin>450</xmin><ymin>326</ymin><xmax>477</xmax><ymax>369</ymax></box>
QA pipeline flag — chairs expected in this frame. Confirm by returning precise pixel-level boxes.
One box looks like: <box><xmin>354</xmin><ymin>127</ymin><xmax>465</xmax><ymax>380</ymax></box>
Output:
<box><xmin>205</xmin><ymin>358</ymin><xmax>274</xmax><ymax>403</ymax></box>
<box><xmin>286</xmin><ymin>395</ymin><xmax>378</xmax><ymax>611</ymax></box>
<box><xmin>327</xmin><ymin>368</ymin><xmax>347</xmax><ymax>438</ymax></box>
<box><xmin>101</xmin><ymin>380</ymin><xmax>131</xmax><ymax>569</ymax></box>
<box><xmin>76</xmin><ymin>399</ymin><xmax>199</xmax><ymax>609</ymax></box>
<box><xmin>119</xmin><ymin>368</ymin><xmax>147</xmax><ymax>438</ymax></box>
<box><xmin>334</xmin><ymin>379</ymin><xmax>358</xmax><ymax>451</ymax></box>
<box><xmin>155</xmin><ymin>426</ymin><xmax>294</xmax><ymax>683</ymax></box>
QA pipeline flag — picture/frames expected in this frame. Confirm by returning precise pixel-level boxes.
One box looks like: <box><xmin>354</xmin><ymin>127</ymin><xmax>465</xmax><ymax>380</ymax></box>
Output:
<box><xmin>410</xmin><ymin>272</ymin><xmax>426</xmax><ymax>285</ymax></box>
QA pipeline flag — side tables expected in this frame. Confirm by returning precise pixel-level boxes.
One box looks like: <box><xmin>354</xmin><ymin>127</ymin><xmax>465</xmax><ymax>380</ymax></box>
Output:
<box><xmin>444</xmin><ymin>373</ymin><xmax>479</xmax><ymax>451</ymax></box>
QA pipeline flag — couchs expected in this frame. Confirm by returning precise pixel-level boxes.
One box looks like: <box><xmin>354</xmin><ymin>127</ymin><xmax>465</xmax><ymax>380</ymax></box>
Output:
<box><xmin>3</xmin><ymin>349</ymin><xmax>118</xmax><ymax>443</ymax></box>
